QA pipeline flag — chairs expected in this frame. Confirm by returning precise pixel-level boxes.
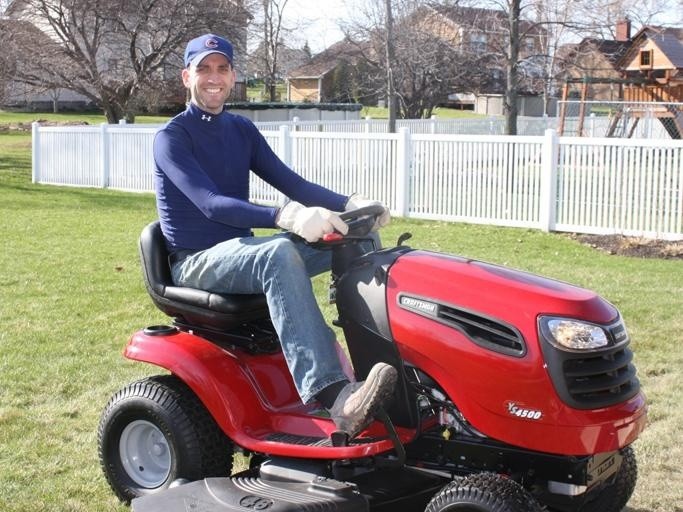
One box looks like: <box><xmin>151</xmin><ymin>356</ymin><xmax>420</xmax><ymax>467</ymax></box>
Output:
<box><xmin>137</xmin><ymin>221</ymin><xmax>270</xmax><ymax>329</ymax></box>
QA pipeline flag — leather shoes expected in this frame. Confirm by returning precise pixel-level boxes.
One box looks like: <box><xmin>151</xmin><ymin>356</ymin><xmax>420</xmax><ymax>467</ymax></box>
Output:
<box><xmin>325</xmin><ymin>361</ymin><xmax>399</xmax><ymax>440</ymax></box>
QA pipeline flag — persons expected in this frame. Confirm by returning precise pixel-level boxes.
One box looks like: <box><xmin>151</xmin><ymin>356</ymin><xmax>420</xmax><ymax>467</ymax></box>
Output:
<box><xmin>150</xmin><ymin>31</ymin><xmax>403</xmax><ymax>442</ymax></box>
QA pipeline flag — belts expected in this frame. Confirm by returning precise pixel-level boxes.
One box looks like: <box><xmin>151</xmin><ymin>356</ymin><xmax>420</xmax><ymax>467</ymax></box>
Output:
<box><xmin>171</xmin><ymin>249</ymin><xmax>191</xmax><ymax>263</ymax></box>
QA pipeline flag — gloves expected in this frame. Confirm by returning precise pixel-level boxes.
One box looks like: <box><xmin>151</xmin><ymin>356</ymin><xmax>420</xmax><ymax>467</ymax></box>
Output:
<box><xmin>275</xmin><ymin>200</ymin><xmax>349</xmax><ymax>243</ymax></box>
<box><xmin>345</xmin><ymin>192</ymin><xmax>390</xmax><ymax>232</ymax></box>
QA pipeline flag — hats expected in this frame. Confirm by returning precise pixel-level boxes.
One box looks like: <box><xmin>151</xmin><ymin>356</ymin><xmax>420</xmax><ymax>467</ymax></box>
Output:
<box><xmin>184</xmin><ymin>34</ymin><xmax>234</xmax><ymax>69</ymax></box>
